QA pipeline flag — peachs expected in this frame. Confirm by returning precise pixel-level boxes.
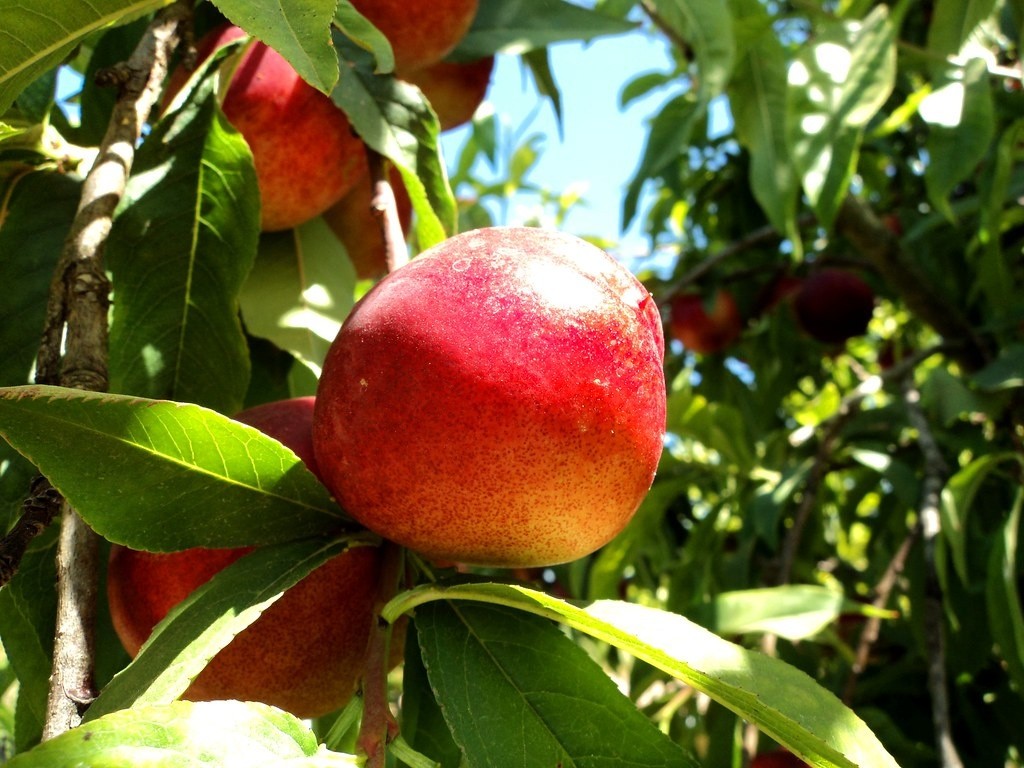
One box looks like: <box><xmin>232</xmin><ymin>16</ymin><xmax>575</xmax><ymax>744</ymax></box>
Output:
<box><xmin>104</xmin><ymin>0</ymin><xmax>906</xmax><ymax>714</ymax></box>
